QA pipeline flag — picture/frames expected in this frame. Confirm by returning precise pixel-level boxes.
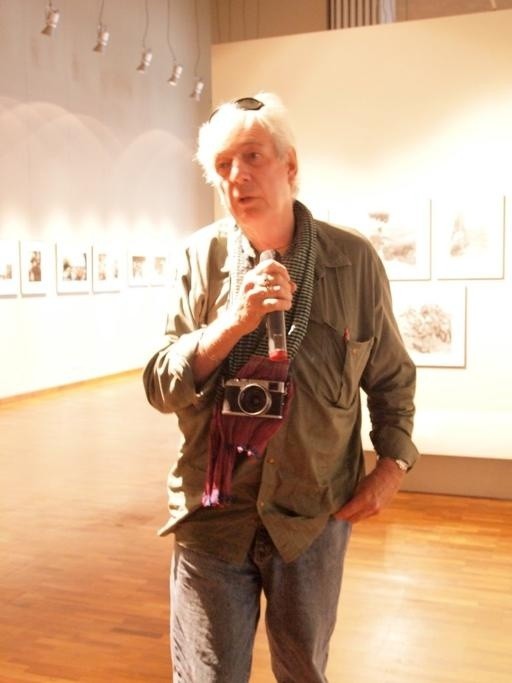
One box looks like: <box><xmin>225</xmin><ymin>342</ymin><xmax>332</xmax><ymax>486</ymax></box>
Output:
<box><xmin>1</xmin><ymin>237</ymin><xmax>179</xmax><ymax>296</ymax></box>
<box><xmin>341</xmin><ymin>193</ymin><xmax>503</xmax><ymax>367</ymax></box>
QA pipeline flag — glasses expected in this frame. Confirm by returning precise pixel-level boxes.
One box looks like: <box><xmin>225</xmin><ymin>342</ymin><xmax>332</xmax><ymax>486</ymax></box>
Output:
<box><xmin>207</xmin><ymin>97</ymin><xmax>263</xmax><ymax>123</ymax></box>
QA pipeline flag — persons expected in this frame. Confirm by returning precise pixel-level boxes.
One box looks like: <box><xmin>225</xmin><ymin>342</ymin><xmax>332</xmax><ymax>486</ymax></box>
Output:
<box><xmin>137</xmin><ymin>86</ymin><xmax>420</xmax><ymax>683</ymax></box>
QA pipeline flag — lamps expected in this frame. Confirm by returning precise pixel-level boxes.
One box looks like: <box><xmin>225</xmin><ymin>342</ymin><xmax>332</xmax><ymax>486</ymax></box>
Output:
<box><xmin>39</xmin><ymin>1</ymin><xmax>206</xmax><ymax>107</ymax></box>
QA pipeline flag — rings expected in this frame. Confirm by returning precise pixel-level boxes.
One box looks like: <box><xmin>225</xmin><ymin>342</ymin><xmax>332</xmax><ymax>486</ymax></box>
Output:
<box><xmin>262</xmin><ymin>273</ymin><xmax>269</xmax><ymax>286</ymax></box>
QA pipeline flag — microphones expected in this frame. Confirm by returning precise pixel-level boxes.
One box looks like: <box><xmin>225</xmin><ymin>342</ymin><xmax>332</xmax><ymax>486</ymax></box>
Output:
<box><xmin>260</xmin><ymin>249</ymin><xmax>288</xmax><ymax>365</ymax></box>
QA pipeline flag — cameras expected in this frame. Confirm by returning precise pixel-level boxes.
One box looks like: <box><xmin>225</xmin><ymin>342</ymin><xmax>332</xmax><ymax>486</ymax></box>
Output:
<box><xmin>220</xmin><ymin>376</ymin><xmax>285</xmax><ymax>422</ymax></box>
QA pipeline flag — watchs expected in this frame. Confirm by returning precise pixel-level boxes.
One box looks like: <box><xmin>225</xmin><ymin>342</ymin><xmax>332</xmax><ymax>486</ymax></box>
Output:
<box><xmin>392</xmin><ymin>456</ymin><xmax>410</xmax><ymax>473</ymax></box>
